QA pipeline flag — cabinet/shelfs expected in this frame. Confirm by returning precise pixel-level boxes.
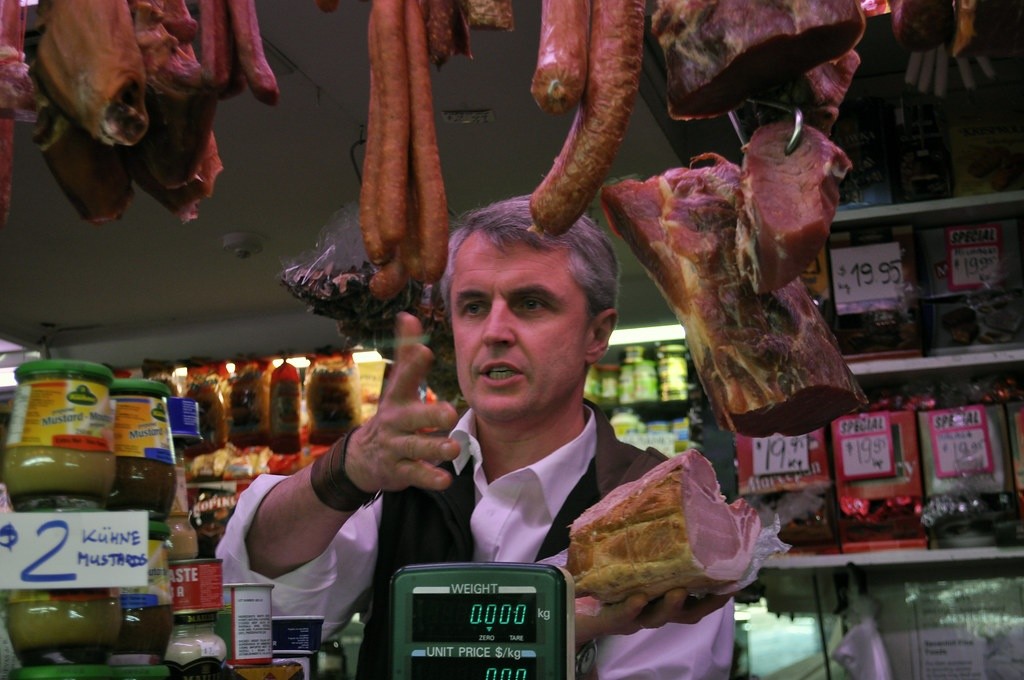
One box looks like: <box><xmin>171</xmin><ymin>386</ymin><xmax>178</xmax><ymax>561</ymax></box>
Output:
<box><xmin>706</xmin><ymin>186</ymin><xmax>1024</xmax><ymax>577</ymax></box>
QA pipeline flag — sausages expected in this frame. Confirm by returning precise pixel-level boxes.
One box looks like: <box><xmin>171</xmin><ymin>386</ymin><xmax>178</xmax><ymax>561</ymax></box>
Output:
<box><xmin>196</xmin><ymin>0</ymin><xmax>644</xmax><ymax>303</ymax></box>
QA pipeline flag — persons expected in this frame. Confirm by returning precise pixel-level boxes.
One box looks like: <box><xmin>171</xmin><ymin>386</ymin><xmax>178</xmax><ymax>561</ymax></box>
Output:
<box><xmin>212</xmin><ymin>195</ymin><xmax>735</xmax><ymax>680</ymax></box>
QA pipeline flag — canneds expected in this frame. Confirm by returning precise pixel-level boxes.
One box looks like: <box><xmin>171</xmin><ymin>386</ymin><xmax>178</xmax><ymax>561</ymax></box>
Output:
<box><xmin>2</xmin><ymin>358</ymin><xmax>273</xmax><ymax>669</ymax></box>
